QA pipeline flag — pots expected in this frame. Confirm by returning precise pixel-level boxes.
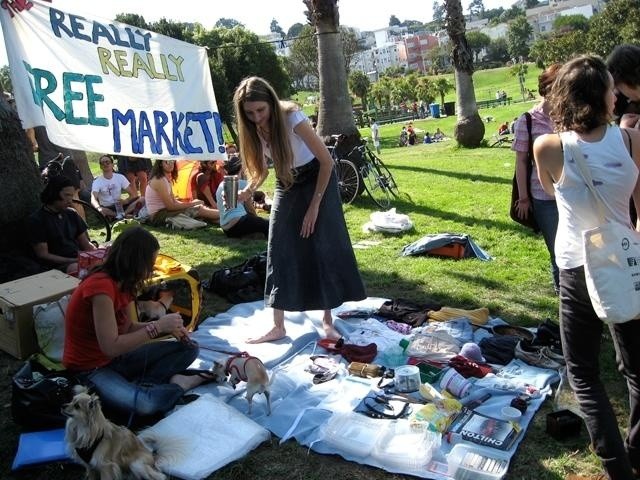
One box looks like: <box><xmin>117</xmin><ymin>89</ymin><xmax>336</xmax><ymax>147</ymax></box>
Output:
<box><xmin>468</xmin><ymin>321</ymin><xmax>534</xmax><ymax>344</ymax></box>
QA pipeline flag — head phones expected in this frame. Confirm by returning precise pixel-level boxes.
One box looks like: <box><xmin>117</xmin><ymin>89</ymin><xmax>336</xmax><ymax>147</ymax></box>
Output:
<box><xmin>38</xmin><ymin>187</ymin><xmax>56</xmax><ymax>205</ymax></box>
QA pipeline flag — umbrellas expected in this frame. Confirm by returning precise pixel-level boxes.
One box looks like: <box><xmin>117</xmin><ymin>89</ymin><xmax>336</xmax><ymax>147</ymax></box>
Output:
<box><xmin>171</xmin><ymin>157</ymin><xmax>224</xmax><ymax>202</ymax></box>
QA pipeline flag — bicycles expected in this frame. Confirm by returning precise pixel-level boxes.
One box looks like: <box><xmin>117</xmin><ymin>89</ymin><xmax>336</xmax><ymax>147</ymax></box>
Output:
<box><xmin>318</xmin><ymin>134</ymin><xmax>360</xmax><ymax>206</ymax></box>
<box><xmin>41</xmin><ymin>151</ymin><xmax>112</xmax><ymax>245</ymax></box>
<box><xmin>348</xmin><ymin>133</ymin><xmax>401</xmax><ymax>210</ymax></box>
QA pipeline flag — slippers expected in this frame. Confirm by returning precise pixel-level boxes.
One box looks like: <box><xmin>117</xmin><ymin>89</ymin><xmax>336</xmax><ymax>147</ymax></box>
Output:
<box><xmin>179</xmin><ymin>370</ymin><xmax>216</xmax><ymax>384</ymax></box>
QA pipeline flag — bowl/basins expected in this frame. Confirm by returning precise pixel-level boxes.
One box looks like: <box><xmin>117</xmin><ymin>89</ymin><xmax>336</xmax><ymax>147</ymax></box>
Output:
<box><xmin>501</xmin><ymin>406</ymin><xmax>522</xmax><ymax>423</ymax></box>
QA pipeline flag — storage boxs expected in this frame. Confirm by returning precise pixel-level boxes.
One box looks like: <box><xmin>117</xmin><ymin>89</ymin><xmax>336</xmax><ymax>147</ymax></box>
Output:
<box><xmin>0</xmin><ymin>268</ymin><xmax>82</xmax><ymax>360</ymax></box>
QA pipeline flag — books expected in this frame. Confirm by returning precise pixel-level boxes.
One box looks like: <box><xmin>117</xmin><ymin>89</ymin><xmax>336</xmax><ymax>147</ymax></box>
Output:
<box><xmin>451</xmin><ymin>407</ymin><xmax>519</xmax><ymax>450</ymax></box>
<box><xmin>455</xmin><ymin>452</ymin><xmax>510</xmax><ymax>480</ymax></box>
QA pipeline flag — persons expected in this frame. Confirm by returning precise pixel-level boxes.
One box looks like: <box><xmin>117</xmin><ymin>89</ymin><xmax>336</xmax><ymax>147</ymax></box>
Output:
<box><xmin>27</xmin><ymin>127</ymin><xmax>87</xmax><ymax>225</ymax></box>
<box><xmin>511</xmin><ymin>64</ymin><xmax>568</xmax><ymax>294</ymax></box>
<box><xmin>215</xmin><ymin>156</ymin><xmax>268</xmax><ymax>239</ymax></box>
<box><xmin>64</xmin><ymin>226</ymin><xmax>226</xmax><ymax>419</ymax></box>
<box><xmin>113</xmin><ymin>153</ymin><xmax>151</xmax><ymax>200</ymax></box>
<box><xmin>192</xmin><ymin>160</ymin><xmax>224</xmax><ymax>208</ymax></box>
<box><xmin>225</xmin><ymin>144</ymin><xmax>239</xmax><ymax>159</ymax></box>
<box><xmin>145</xmin><ymin>160</ymin><xmax>220</xmax><ymax>225</ymax></box>
<box><xmin>369</xmin><ymin>117</ymin><xmax>380</xmax><ymax>154</ymax></box>
<box><xmin>397</xmin><ymin>98</ymin><xmax>430</xmax><ymax>121</ymax></box>
<box><xmin>511</xmin><ymin>117</ymin><xmax>518</xmax><ymax>133</ymax></box>
<box><xmin>533</xmin><ymin>52</ymin><xmax>640</xmax><ymax>480</ymax></box>
<box><xmin>499</xmin><ymin>121</ymin><xmax>510</xmax><ymax>134</ymax></box>
<box><xmin>496</xmin><ymin>90</ymin><xmax>507</xmax><ymax>105</ymax></box>
<box><xmin>598</xmin><ymin>46</ymin><xmax>639</xmax><ymax>130</ymax></box>
<box><xmin>34</xmin><ymin>174</ymin><xmax>99</xmax><ymax>273</ymax></box>
<box><xmin>90</xmin><ymin>154</ymin><xmax>146</xmax><ymax>220</ymax></box>
<box><xmin>423</xmin><ymin>132</ymin><xmax>432</xmax><ymax>144</ymax></box>
<box><xmin>232</xmin><ymin>76</ymin><xmax>368</xmax><ymax>346</ymax></box>
<box><xmin>400</xmin><ymin>122</ymin><xmax>418</xmax><ymax>146</ymax></box>
<box><xmin>432</xmin><ymin>128</ymin><xmax>445</xmax><ymax>142</ymax></box>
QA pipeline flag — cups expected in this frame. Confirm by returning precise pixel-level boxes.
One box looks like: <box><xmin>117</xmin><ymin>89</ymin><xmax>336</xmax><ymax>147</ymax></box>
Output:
<box><xmin>439</xmin><ymin>367</ymin><xmax>472</xmax><ymax>399</ymax></box>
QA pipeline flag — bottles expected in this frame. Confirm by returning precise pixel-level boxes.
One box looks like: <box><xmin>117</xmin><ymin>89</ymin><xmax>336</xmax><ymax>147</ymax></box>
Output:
<box><xmin>224</xmin><ymin>174</ymin><xmax>239</xmax><ymax>208</ymax></box>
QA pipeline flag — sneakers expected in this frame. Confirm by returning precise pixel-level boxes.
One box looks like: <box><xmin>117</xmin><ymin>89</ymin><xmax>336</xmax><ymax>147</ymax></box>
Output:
<box><xmin>566</xmin><ymin>473</ymin><xmax>610</xmax><ymax>480</ymax></box>
<box><xmin>534</xmin><ymin>346</ymin><xmax>565</xmax><ymax>364</ymax></box>
<box><xmin>515</xmin><ymin>341</ymin><xmax>559</xmax><ymax>369</ymax></box>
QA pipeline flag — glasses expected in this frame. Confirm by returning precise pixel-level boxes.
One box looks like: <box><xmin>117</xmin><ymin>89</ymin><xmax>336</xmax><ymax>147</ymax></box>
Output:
<box><xmin>166</xmin><ymin>160</ymin><xmax>175</xmax><ymax>165</ymax></box>
<box><xmin>100</xmin><ymin>161</ymin><xmax>110</xmax><ymax>165</ymax></box>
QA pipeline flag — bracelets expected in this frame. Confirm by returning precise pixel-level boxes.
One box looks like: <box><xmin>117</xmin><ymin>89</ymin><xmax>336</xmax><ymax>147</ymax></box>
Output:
<box><xmin>514</xmin><ymin>197</ymin><xmax>530</xmax><ymax>205</ymax></box>
<box><xmin>146</xmin><ymin>323</ymin><xmax>158</xmax><ymax>340</ymax></box>
<box><xmin>314</xmin><ymin>192</ymin><xmax>323</xmax><ymax>200</ymax></box>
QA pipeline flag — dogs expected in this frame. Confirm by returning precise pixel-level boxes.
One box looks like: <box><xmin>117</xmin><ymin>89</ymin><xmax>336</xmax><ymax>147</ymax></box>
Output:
<box><xmin>59</xmin><ymin>384</ymin><xmax>167</xmax><ymax>480</ymax></box>
<box><xmin>212</xmin><ymin>353</ymin><xmax>272</xmax><ymax>417</ymax></box>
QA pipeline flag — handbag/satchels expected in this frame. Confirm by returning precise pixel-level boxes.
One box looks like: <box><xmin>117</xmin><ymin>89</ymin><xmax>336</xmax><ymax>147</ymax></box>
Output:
<box><xmin>510</xmin><ymin>160</ymin><xmax>539</xmax><ymax>232</ymax></box>
<box><xmin>164</xmin><ymin>213</ymin><xmax>207</xmax><ymax>230</ymax></box>
<box><xmin>583</xmin><ymin>224</ymin><xmax>640</xmax><ymax>325</ymax></box>
<box><xmin>9</xmin><ymin>358</ymin><xmax>80</xmax><ymax>429</ymax></box>
<box><xmin>211</xmin><ymin>252</ymin><xmax>267</xmax><ymax>303</ymax></box>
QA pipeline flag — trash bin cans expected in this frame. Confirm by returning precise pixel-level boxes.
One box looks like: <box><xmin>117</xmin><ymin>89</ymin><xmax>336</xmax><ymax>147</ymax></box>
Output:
<box><xmin>429</xmin><ymin>103</ymin><xmax>440</xmax><ymax>117</ymax></box>
<box><xmin>444</xmin><ymin>101</ymin><xmax>456</xmax><ymax>115</ymax></box>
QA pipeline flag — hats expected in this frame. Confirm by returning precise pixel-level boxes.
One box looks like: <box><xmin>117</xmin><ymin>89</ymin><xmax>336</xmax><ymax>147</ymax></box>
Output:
<box><xmin>47</xmin><ymin>152</ymin><xmax>81</xmax><ymax>188</ymax></box>
<box><xmin>223</xmin><ymin>157</ymin><xmax>242</xmax><ymax>174</ymax></box>
<box><xmin>460</xmin><ymin>343</ymin><xmax>487</xmax><ymax>364</ymax></box>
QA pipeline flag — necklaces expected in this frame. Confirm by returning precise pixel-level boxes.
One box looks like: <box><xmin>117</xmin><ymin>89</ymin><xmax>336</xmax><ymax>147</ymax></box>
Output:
<box><xmin>259</xmin><ymin>126</ymin><xmax>276</xmax><ymax>148</ymax></box>
